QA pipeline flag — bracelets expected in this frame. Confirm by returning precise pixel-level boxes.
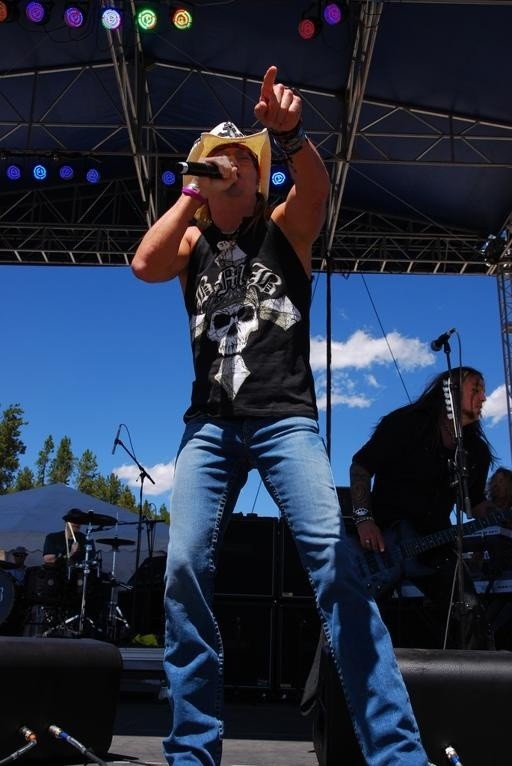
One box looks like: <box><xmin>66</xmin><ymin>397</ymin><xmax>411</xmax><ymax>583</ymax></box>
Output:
<box><xmin>352</xmin><ymin>506</ymin><xmax>375</xmax><ymax>527</ymax></box>
<box><xmin>269</xmin><ymin>121</ymin><xmax>307</xmax><ymax>156</ymax></box>
<box><xmin>179</xmin><ymin>183</ymin><xmax>207</xmax><ymax>204</ymax></box>
<box><xmin>57</xmin><ymin>551</ymin><xmax>75</xmax><ymax>564</ymax></box>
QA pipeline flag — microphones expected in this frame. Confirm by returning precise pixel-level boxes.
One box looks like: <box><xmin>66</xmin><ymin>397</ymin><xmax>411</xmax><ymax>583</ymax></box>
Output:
<box><xmin>172</xmin><ymin>159</ymin><xmax>221</xmax><ymax>179</ymax></box>
<box><xmin>430</xmin><ymin>327</ymin><xmax>457</xmax><ymax>353</ymax></box>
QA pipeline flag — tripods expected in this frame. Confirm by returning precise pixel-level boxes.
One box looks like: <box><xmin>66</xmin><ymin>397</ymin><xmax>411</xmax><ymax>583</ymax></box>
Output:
<box><xmin>34</xmin><ymin>520</ymin><xmax>140</xmax><ymax>643</ymax></box>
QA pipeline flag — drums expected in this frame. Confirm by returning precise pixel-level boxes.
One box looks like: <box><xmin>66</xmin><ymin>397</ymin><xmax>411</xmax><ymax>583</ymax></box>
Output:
<box><xmin>0</xmin><ymin>571</ymin><xmax>23</xmax><ymax>632</ymax></box>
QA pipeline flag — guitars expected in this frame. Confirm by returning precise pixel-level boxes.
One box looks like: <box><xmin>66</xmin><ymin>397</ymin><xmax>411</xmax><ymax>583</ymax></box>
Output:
<box><xmin>345</xmin><ymin>509</ymin><xmax>510</xmax><ymax>601</ymax></box>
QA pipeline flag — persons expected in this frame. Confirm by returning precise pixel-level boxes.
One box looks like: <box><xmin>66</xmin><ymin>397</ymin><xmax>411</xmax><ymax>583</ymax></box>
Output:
<box><xmin>130</xmin><ymin>63</ymin><xmax>431</xmax><ymax>766</ymax></box>
<box><xmin>8</xmin><ymin>546</ymin><xmax>29</xmax><ymax>581</ymax></box>
<box><xmin>298</xmin><ymin>367</ymin><xmax>497</xmax><ymax>716</ymax></box>
<box><xmin>43</xmin><ymin>508</ymin><xmax>98</xmax><ymax>621</ymax></box>
<box><xmin>486</xmin><ymin>467</ymin><xmax>512</xmax><ymax>528</ymax></box>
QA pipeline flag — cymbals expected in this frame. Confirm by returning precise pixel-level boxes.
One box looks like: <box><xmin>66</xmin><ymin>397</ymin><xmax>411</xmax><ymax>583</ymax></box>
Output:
<box><xmin>60</xmin><ymin>510</ymin><xmax>117</xmax><ymax>527</ymax></box>
<box><xmin>96</xmin><ymin>537</ymin><xmax>135</xmax><ymax>546</ymax></box>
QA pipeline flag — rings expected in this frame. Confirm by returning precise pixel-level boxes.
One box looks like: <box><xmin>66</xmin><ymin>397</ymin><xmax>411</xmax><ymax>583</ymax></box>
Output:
<box><xmin>364</xmin><ymin>539</ymin><xmax>371</xmax><ymax>544</ymax></box>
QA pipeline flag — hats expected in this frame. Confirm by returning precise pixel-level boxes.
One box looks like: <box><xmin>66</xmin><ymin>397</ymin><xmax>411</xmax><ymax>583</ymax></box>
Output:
<box><xmin>11</xmin><ymin>546</ymin><xmax>28</xmax><ymax>556</ymax></box>
<box><xmin>182</xmin><ymin>120</ymin><xmax>271</xmax><ymax>222</ymax></box>
